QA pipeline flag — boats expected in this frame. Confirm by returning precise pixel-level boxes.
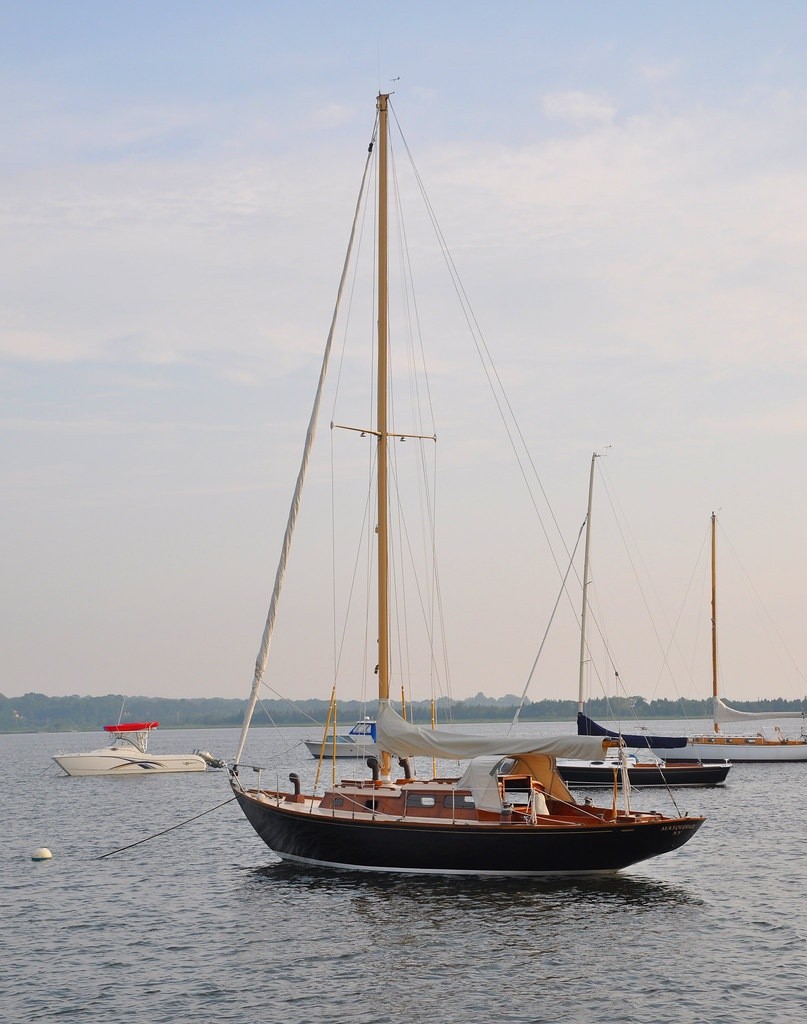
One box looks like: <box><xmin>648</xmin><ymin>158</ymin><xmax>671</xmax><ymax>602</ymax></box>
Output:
<box><xmin>302</xmin><ymin>717</ymin><xmax>399</xmax><ymax>757</ymax></box>
<box><xmin>50</xmin><ymin>720</ymin><xmax>229</xmax><ymax>776</ymax></box>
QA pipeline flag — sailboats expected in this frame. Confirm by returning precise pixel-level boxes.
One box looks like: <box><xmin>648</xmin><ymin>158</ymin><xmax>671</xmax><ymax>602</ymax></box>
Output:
<box><xmin>575</xmin><ymin>506</ymin><xmax>807</xmax><ymax>763</ymax></box>
<box><xmin>472</xmin><ymin>446</ymin><xmax>734</xmax><ymax>790</ymax></box>
<box><xmin>222</xmin><ymin>88</ymin><xmax>706</xmax><ymax>876</ymax></box>
